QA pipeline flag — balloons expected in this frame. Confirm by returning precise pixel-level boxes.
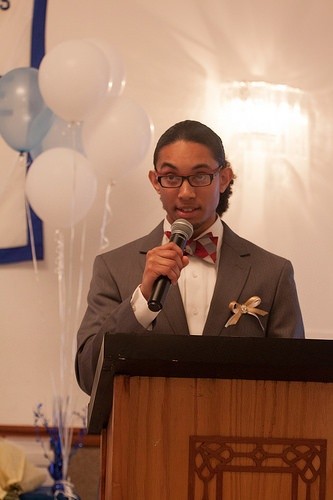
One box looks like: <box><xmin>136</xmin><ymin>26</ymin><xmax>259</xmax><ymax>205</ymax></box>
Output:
<box><xmin>0</xmin><ymin>37</ymin><xmax>155</xmax><ymax>230</ymax></box>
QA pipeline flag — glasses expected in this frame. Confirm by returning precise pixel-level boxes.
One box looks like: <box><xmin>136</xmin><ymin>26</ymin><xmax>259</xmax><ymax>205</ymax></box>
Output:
<box><xmin>153</xmin><ymin>163</ymin><xmax>225</xmax><ymax>188</ymax></box>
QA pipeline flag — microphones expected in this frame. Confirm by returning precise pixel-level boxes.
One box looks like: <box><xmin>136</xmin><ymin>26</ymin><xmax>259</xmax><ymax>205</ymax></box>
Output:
<box><xmin>149</xmin><ymin>219</ymin><xmax>193</xmax><ymax>312</ymax></box>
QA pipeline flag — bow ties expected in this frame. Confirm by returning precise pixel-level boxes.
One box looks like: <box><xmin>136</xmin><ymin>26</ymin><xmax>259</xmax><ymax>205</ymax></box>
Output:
<box><xmin>165</xmin><ymin>230</ymin><xmax>218</xmax><ymax>266</ymax></box>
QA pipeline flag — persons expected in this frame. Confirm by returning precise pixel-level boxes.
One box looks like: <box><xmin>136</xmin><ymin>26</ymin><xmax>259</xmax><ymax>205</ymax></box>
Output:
<box><xmin>75</xmin><ymin>120</ymin><xmax>305</xmax><ymax>396</ymax></box>
